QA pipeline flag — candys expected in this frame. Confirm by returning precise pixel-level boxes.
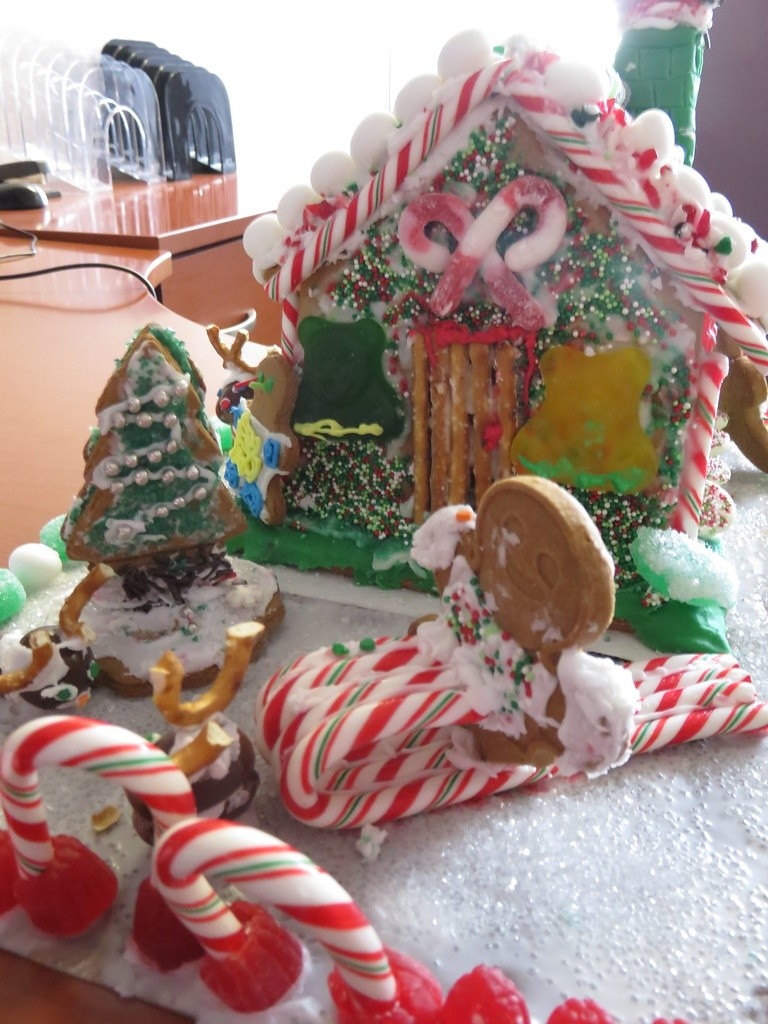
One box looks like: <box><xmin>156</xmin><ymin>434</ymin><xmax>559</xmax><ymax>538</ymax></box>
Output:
<box><xmin>0</xmin><ymin>1</ymin><xmax>768</xmax><ymax>1023</ymax></box>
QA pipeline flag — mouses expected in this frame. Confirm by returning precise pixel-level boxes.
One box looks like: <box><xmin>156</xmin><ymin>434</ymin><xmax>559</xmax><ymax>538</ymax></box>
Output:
<box><xmin>0</xmin><ymin>182</ymin><xmax>50</xmax><ymax>210</ymax></box>
